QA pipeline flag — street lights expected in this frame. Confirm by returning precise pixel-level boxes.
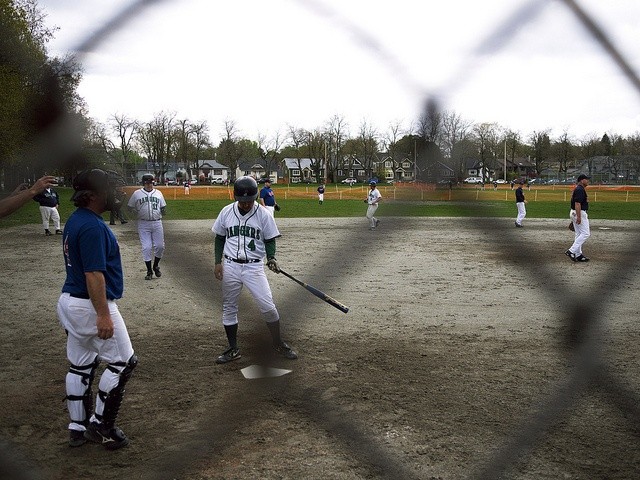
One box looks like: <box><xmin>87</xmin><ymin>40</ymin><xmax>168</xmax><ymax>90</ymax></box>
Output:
<box><xmin>411</xmin><ymin>134</ymin><xmax>419</xmax><ymax>182</ymax></box>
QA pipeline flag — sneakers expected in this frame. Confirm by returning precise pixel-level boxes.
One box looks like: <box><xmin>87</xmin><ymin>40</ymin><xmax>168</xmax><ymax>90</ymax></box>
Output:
<box><xmin>565</xmin><ymin>250</ymin><xmax>578</xmax><ymax>262</ymax></box>
<box><xmin>576</xmin><ymin>254</ymin><xmax>590</xmax><ymax>262</ymax></box>
<box><xmin>46</xmin><ymin>231</ymin><xmax>53</xmax><ymax>235</ymax></box>
<box><xmin>215</xmin><ymin>347</ymin><xmax>242</xmax><ymax>364</ymax></box>
<box><xmin>84</xmin><ymin>421</ymin><xmax>129</xmax><ymax>449</ymax></box>
<box><xmin>375</xmin><ymin>220</ymin><xmax>379</xmax><ymax>226</ymax></box>
<box><xmin>56</xmin><ymin>230</ymin><xmax>63</xmax><ymax>234</ymax></box>
<box><xmin>145</xmin><ymin>271</ymin><xmax>153</xmax><ymax>280</ymax></box>
<box><xmin>70</xmin><ymin>429</ymin><xmax>87</xmax><ymax>447</ymax></box>
<box><xmin>369</xmin><ymin>227</ymin><xmax>375</xmax><ymax>231</ymax></box>
<box><xmin>515</xmin><ymin>222</ymin><xmax>522</xmax><ymax>228</ymax></box>
<box><xmin>153</xmin><ymin>267</ymin><xmax>162</xmax><ymax>277</ymax></box>
<box><xmin>273</xmin><ymin>341</ymin><xmax>298</xmax><ymax>359</ymax></box>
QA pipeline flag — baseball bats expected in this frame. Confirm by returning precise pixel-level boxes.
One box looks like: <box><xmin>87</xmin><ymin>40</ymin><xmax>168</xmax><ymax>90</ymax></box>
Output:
<box><xmin>267</xmin><ymin>262</ymin><xmax>349</xmax><ymax>313</ymax></box>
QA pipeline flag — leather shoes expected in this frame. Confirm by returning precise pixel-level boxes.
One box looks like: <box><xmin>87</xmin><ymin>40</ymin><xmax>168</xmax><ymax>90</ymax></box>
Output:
<box><xmin>110</xmin><ymin>223</ymin><xmax>116</xmax><ymax>225</ymax></box>
<box><xmin>122</xmin><ymin>221</ymin><xmax>127</xmax><ymax>224</ymax></box>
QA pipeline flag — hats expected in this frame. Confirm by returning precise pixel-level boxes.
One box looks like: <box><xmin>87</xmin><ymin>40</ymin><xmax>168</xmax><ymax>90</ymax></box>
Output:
<box><xmin>577</xmin><ymin>174</ymin><xmax>591</xmax><ymax>182</ymax></box>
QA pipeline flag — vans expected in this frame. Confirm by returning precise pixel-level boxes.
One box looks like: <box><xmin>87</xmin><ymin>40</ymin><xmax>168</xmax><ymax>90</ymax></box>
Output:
<box><xmin>206</xmin><ymin>177</ymin><xmax>217</xmax><ymax>183</ymax></box>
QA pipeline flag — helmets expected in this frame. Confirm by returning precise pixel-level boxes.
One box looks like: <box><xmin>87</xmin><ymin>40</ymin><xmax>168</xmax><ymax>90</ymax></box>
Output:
<box><xmin>264</xmin><ymin>179</ymin><xmax>271</xmax><ymax>182</ymax></box>
<box><xmin>142</xmin><ymin>173</ymin><xmax>155</xmax><ymax>184</ymax></box>
<box><xmin>234</xmin><ymin>176</ymin><xmax>258</xmax><ymax>201</ymax></box>
<box><xmin>73</xmin><ymin>169</ymin><xmax>126</xmax><ymax>211</ymax></box>
<box><xmin>369</xmin><ymin>181</ymin><xmax>376</xmax><ymax>186</ymax></box>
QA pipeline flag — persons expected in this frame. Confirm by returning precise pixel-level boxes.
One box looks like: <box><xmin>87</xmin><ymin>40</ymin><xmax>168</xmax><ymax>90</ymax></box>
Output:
<box><xmin>514</xmin><ymin>182</ymin><xmax>528</xmax><ymax>227</ymax></box>
<box><xmin>209</xmin><ymin>177</ymin><xmax>299</xmax><ymax>363</ymax></box>
<box><xmin>33</xmin><ymin>187</ymin><xmax>63</xmax><ymax>235</ymax></box>
<box><xmin>364</xmin><ymin>181</ymin><xmax>382</xmax><ymax>231</ymax></box>
<box><xmin>110</xmin><ymin>210</ymin><xmax>128</xmax><ymax>225</ymax></box>
<box><xmin>127</xmin><ymin>174</ymin><xmax>168</xmax><ymax>280</ymax></box>
<box><xmin>0</xmin><ymin>175</ymin><xmax>60</xmax><ymax>220</ymax></box>
<box><xmin>260</xmin><ymin>179</ymin><xmax>278</xmax><ymax>219</ymax></box>
<box><xmin>563</xmin><ymin>174</ymin><xmax>592</xmax><ymax>263</ymax></box>
<box><xmin>184</xmin><ymin>181</ymin><xmax>190</xmax><ymax>195</ymax></box>
<box><xmin>57</xmin><ymin>169</ymin><xmax>137</xmax><ymax>451</ymax></box>
<box><xmin>317</xmin><ymin>185</ymin><xmax>324</xmax><ymax>205</ymax></box>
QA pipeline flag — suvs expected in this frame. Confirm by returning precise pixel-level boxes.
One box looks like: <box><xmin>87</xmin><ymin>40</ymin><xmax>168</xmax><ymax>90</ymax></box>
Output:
<box><xmin>341</xmin><ymin>177</ymin><xmax>358</xmax><ymax>185</ymax></box>
<box><xmin>212</xmin><ymin>177</ymin><xmax>225</xmax><ymax>185</ymax></box>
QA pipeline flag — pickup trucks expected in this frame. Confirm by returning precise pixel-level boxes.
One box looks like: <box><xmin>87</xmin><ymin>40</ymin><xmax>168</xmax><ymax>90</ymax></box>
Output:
<box><xmin>464</xmin><ymin>177</ymin><xmax>482</xmax><ymax>185</ymax></box>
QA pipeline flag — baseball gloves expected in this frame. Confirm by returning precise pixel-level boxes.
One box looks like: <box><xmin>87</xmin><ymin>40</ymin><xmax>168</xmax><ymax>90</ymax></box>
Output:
<box><xmin>275</xmin><ymin>205</ymin><xmax>281</xmax><ymax>211</ymax></box>
<box><xmin>569</xmin><ymin>222</ymin><xmax>574</xmax><ymax>231</ymax></box>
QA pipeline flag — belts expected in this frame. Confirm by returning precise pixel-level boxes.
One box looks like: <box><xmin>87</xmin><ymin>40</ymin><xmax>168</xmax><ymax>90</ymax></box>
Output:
<box><xmin>70</xmin><ymin>292</ymin><xmax>90</xmax><ymax>299</ymax></box>
<box><xmin>141</xmin><ymin>218</ymin><xmax>162</xmax><ymax>221</ymax></box>
<box><xmin>224</xmin><ymin>255</ymin><xmax>261</xmax><ymax>263</ymax></box>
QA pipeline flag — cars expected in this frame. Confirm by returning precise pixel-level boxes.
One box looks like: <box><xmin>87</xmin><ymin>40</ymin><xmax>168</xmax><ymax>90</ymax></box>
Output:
<box><xmin>492</xmin><ymin>179</ymin><xmax>508</xmax><ymax>184</ymax></box>
<box><xmin>528</xmin><ymin>179</ymin><xmax>539</xmax><ymax>185</ymax></box>
<box><xmin>166</xmin><ymin>177</ymin><xmax>176</xmax><ymax>185</ymax></box>
<box><xmin>182</xmin><ymin>179</ymin><xmax>197</xmax><ymax>185</ymax></box>
<box><xmin>409</xmin><ymin>179</ymin><xmax>423</xmax><ymax>185</ymax></box>
<box><xmin>386</xmin><ymin>179</ymin><xmax>401</xmax><ymax>184</ymax></box>
